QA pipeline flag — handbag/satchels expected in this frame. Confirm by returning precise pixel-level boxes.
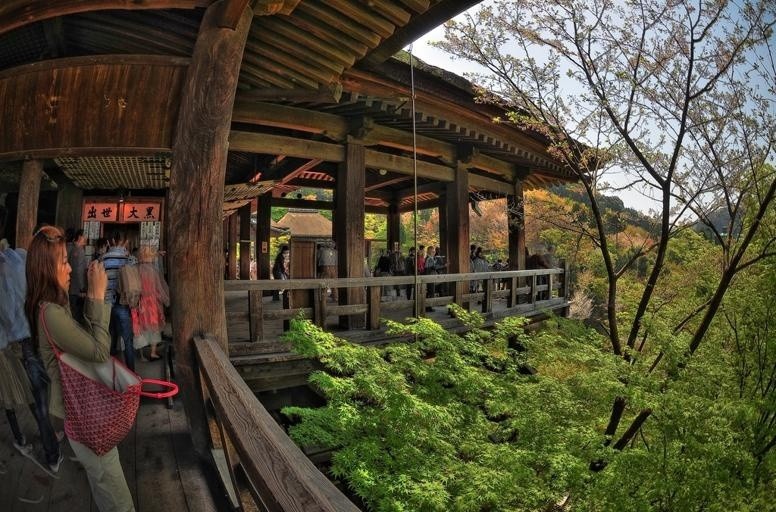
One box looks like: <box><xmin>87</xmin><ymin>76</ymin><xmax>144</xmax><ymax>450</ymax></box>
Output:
<box><xmin>57</xmin><ymin>354</ymin><xmax>142</xmax><ymax>458</ymax></box>
<box><xmin>120</xmin><ymin>266</ymin><xmax>140</xmax><ymax>306</ymax></box>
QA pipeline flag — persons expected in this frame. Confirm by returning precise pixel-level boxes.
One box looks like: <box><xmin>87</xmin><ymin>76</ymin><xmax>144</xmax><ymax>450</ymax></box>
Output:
<box><xmin>70</xmin><ymin>234</ymin><xmax>170</xmax><ymax>372</ymax></box>
<box><xmin>2</xmin><ymin>238</ymin><xmax>64</xmax><ymax>471</ymax></box>
<box><xmin>235</xmin><ymin>244</ymin><xmax>289</xmax><ymax>303</ymax></box>
<box><xmin>316</xmin><ymin>238</ymin><xmax>559</xmax><ymax>309</ymax></box>
<box><xmin>23</xmin><ymin>229</ymin><xmax>139</xmax><ymax>511</ymax></box>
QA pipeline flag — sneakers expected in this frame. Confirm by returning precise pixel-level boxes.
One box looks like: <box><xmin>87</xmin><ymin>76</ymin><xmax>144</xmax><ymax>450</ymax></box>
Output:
<box><xmin>51</xmin><ymin>447</ymin><xmax>64</xmax><ymax>471</ymax></box>
